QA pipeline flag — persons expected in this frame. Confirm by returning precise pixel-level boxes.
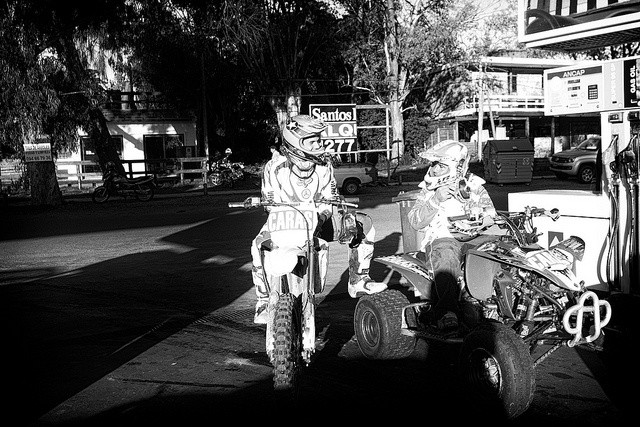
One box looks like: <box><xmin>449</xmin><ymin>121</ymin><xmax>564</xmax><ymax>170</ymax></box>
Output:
<box><xmin>406</xmin><ymin>139</ymin><xmax>498</xmax><ymax>330</ymax></box>
<box><xmin>251</xmin><ymin>115</ymin><xmax>389</xmax><ymax>325</ymax></box>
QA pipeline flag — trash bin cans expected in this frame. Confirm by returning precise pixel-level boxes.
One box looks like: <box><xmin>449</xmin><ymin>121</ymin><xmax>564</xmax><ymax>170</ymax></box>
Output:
<box><xmin>482</xmin><ymin>138</ymin><xmax>534</xmax><ymax>186</ymax></box>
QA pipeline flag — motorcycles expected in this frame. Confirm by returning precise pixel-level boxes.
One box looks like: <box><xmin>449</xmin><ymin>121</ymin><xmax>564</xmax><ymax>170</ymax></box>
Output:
<box><xmin>206</xmin><ymin>148</ymin><xmax>265</xmax><ymax>188</ymax></box>
<box><xmin>93</xmin><ymin>161</ymin><xmax>156</xmax><ymax>202</ymax></box>
<box><xmin>228</xmin><ymin>195</ymin><xmax>358</xmax><ymax>391</ymax></box>
<box><xmin>354</xmin><ymin>205</ymin><xmax>611</xmax><ymax>419</ymax></box>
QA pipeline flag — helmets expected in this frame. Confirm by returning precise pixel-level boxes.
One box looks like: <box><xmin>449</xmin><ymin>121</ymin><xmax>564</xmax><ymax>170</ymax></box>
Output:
<box><xmin>283</xmin><ymin>115</ymin><xmax>329</xmax><ymax>166</ymax></box>
<box><xmin>417</xmin><ymin>140</ymin><xmax>470</xmax><ymax>190</ymax></box>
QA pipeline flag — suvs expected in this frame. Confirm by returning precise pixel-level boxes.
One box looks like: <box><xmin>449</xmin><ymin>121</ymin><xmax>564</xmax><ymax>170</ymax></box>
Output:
<box><xmin>548</xmin><ymin>136</ymin><xmax>599</xmax><ymax>182</ymax></box>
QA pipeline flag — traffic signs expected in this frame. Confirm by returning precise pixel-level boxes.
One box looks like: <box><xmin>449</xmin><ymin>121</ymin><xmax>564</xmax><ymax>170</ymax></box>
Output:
<box><xmin>309</xmin><ymin>103</ymin><xmax>359</xmax><ymax>155</ymax></box>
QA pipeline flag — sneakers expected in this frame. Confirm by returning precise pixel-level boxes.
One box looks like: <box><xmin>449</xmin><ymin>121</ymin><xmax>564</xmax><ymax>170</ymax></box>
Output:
<box><xmin>255</xmin><ymin>297</ymin><xmax>269</xmax><ymax>323</ymax></box>
<box><xmin>348</xmin><ymin>279</ymin><xmax>387</xmax><ymax>299</ymax></box>
<box><xmin>436</xmin><ymin>312</ymin><xmax>457</xmax><ymax>329</ymax></box>
